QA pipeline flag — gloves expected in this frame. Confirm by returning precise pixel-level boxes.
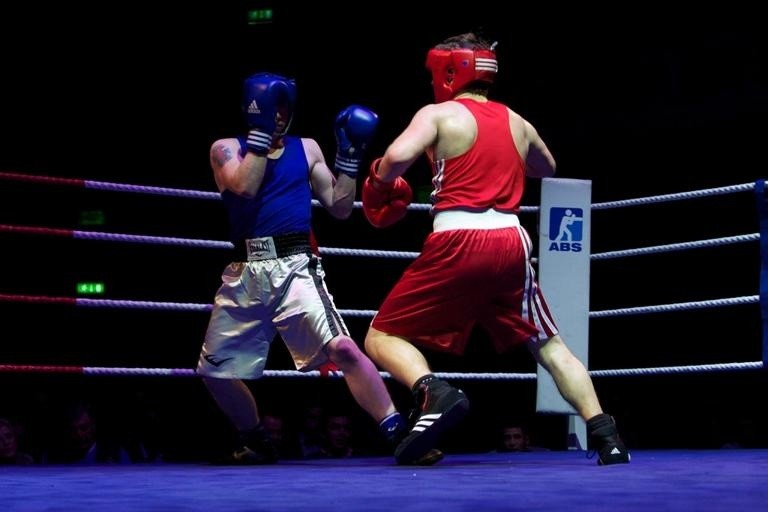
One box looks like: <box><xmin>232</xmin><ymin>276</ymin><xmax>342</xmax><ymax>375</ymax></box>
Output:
<box><xmin>335</xmin><ymin>104</ymin><xmax>378</xmax><ymax>179</ymax></box>
<box><xmin>242</xmin><ymin>73</ymin><xmax>294</xmax><ymax>156</ymax></box>
<box><xmin>362</xmin><ymin>157</ymin><xmax>414</xmax><ymax>229</ymax></box>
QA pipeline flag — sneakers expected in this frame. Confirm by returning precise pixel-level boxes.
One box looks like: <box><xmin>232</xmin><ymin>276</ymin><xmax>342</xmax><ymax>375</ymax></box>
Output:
<box><xmin>585</xmin><ymin>413</ymin><xmax>633</xmax><ymax>465</ymax></box>
<box><xmin>393</xmin><ymin>380</ymin><xmax>469</xmax><ymax>466</ymax></box>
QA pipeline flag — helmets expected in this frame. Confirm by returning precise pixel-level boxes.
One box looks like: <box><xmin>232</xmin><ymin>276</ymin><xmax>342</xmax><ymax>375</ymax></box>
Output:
<box><xmin>426</xmin><ymin>49</ymin><xmax>499</xmax><ymax>102</ymax></box>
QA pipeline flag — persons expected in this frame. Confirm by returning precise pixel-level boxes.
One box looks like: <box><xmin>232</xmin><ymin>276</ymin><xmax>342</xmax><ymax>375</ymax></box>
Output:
<box><xmin>501</xmin><ymin>422</ymin><xmax>531</xmax><ymax>452</ymax></box>
<box><xmin>2</xmin><ymin>409</ymin><xmax>356</xmax><ymax>465</ymax></box>
<box><xmin>194</xmin><ymin>68</ymin><xmax>450</xmax><ymax>466</ymax></box>
<box><xmin>357</xmin><ymin>31</ymin><xmax>632</xmax><ymax>467</ymax></box>
